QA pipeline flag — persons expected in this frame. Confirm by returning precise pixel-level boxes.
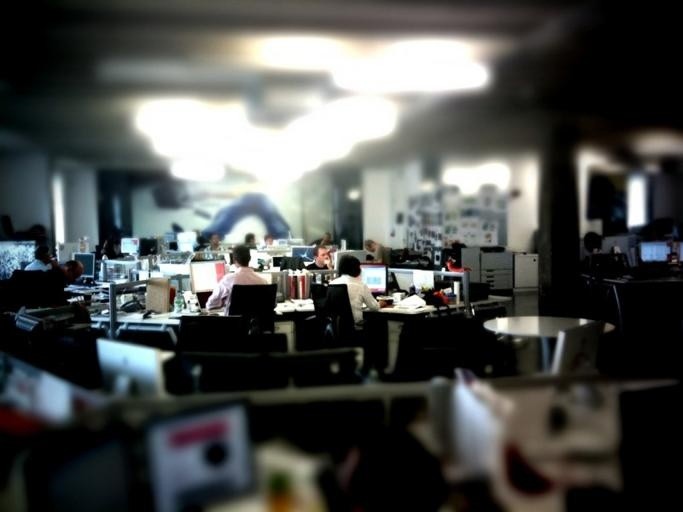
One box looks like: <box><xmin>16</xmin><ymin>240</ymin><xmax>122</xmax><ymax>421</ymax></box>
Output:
<box><xmin>580</xmin><ymin>232</ymin><xmax>617</xmax><ymax>272</ymax></box>
<box><xmin>100</xmin><ymin>223</ymin><xmax>131</xmax><ymax>259</ymax></box>
<box><xmin>193</xmin><ymin>232</ymin><xmax>391</xmax><ymax>378</ymax></box>
<box><xmin>9</xmin><ymin>224</ymin><xmax>91</xmax><ymax>325</ymax></box>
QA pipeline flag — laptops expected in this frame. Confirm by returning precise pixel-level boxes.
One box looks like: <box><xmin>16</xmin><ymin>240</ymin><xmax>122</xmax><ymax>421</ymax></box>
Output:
<box><xmin>196</xmin><ymin>289</ymin><xmax>225</xmax><ymax>313</ymax></box>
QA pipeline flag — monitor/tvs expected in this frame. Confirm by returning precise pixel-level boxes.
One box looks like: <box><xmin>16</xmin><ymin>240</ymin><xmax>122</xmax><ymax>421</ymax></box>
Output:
<box><xmin>190</xmin><ymin>259</ymin><xmax>226</xmax><ymax>293</ymax></box>
<box><xmin>360</xmin><ymin>264</ymin><xmax>387</xmax><ymax>295</ymax></box>
<box><xmin>340</xmin><ymin>237</ymin><xmax>349</xmax><ymax>251</ymax></box>
<box><xmin>72</xmin><ymin>252</ymin><xmax>95</xmax><ymax>280</ymax></box>
<box><xmin>291</xmin><ymin>245</ymin><xmax>314</xmax><ymax>263</ymax></box>
<box><xmin>140</xmin><ymin>397</ymin><xmax>254</xmax><ymax>512</ymax></box>
<box><xmin>1</xmin><ymin>240</ymin><xmax>38</xmax><ymax>280</ymax></box>
<box><xmin>176</xmin><ymin>230</ymin><xmax>198</xmax><ymax>252</ymax></box>
<box><xmin>140</xmin><ymin>238</ymin><xmax>156</xmax><ymax>255</ymax></box>
<box><xmin>95</xmin><ymin>335</ymin><xmax>170</xmax><ymax>396</ymax></box>
<box><xmin>335</xmin><ymin>249</ymin><xmax>366</xmax><ymax>280</ymax></box>
<box><xmin>642</xmin><ymin>242</ymin><xmax>669</xmax><ymax>262</ymax></box>
<box><xmin>120</xmin><ymin>236</ymin><xmax>140</xmax><ymax>252</ymax></box>
